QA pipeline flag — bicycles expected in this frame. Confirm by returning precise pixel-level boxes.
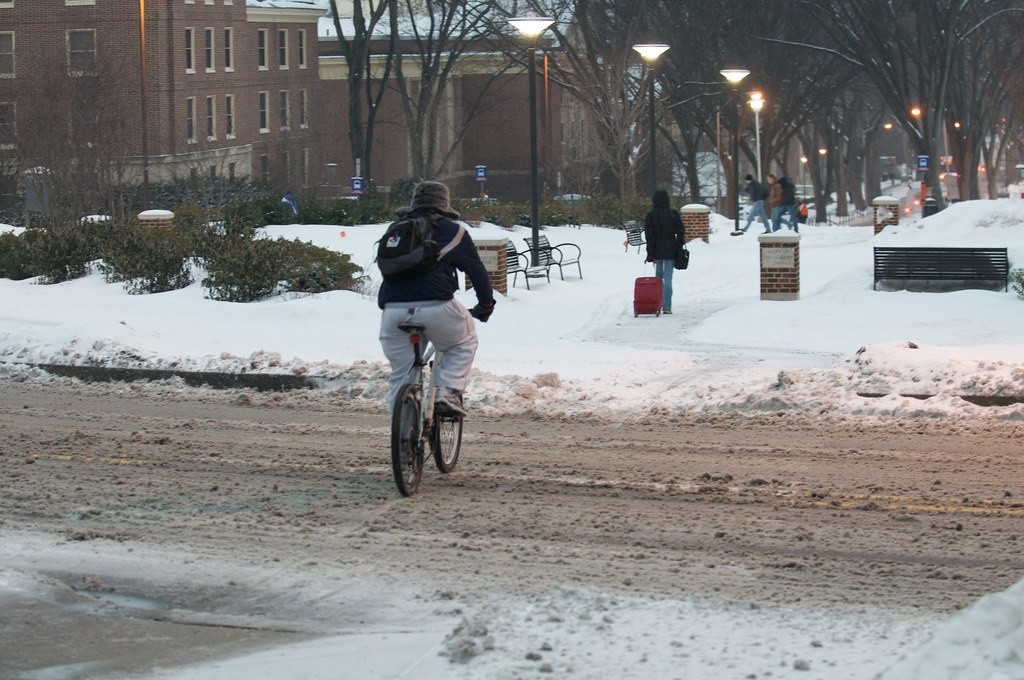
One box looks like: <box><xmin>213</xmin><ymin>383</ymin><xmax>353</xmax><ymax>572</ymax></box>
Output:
<box><xmin>391</xmin><ymin>309</ymin><xmax>492</xmax><ymax>497</ymax></box>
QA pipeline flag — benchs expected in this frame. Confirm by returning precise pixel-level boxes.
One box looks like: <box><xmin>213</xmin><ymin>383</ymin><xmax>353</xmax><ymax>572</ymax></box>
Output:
<box><xmin>873</xmin><ymin>246</ymin><xmax>1010</xmax><ymax>291</ymax></box>
<box><xmin>619</xmin><ymin>221</ymin><xmax>647</xmax><ymax>254</ymax></box>
<box><xmin>523</xmin><ymin>235</ymin><xmax>583</xmax><ymax>285</ymax></box>
<box><xmin>506</xmin><ymin>237</ymin><xmax>551</xmax><ymax>290</ymax></box>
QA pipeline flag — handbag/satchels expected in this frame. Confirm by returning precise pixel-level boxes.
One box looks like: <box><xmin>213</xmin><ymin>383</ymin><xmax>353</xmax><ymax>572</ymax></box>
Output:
<box><xmin>675</xmin><ymin>243</ymin><xmax>689</xmax><ymax>269</ymax></box>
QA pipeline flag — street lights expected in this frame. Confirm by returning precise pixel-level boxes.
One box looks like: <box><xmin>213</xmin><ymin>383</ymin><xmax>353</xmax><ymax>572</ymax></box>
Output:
<box><xmin>506</xmin><ymin>17</ymin><xmax>556</xmax><ymax>267</ymax></box>
<box><xmin>800</xmin><ymin>157</ymin><xmax>807</xmax><ymax>196</ymax></box>
<box><xmin>633</xmin><ymin>44</ymin><xmax>671</xmax><ymax>191</ymax></box>
<box><xmin>819</xmin><ymin>149</ymin><xmax>827</xmax><ymax>179</ymax></box>
<box><xmin>721</xmin><ymin>69</ymin><xmax>750</xmax><ymax>231</ymax></box>
<box><xmin>746</xmin><ymin>92</ymin><xmax>766</xmax><ymax>183</ymax></box>
<box><xmin>862</xmin><ymin>123</ymin><xmax>892</xmax><ymax>206</ymax></box>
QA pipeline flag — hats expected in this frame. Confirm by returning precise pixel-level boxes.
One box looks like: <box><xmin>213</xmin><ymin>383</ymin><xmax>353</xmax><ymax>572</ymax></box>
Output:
<box><xmin>393</xmin><ymin>182</ymin><xmax>460</xmax><ymax>218</ymax></box>
<box><xmin>745</xmin><ymin>175</ymin><xmax>753</xmax><ymax>180</ymax></box>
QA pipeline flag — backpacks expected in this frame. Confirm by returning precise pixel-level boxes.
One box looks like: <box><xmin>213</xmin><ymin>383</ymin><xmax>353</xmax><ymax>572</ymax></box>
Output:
<box><xmin>374</xmin><ymin>212</ymin><xmax>466</xmax><ymax>283</ymax></box>
<box><xmin>758</xmin><ymin>184</ymin><xmax>769</xmax><ymax>200</ymax></box>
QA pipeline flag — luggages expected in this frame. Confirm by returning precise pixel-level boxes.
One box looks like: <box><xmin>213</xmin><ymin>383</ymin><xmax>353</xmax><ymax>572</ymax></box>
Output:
<box><xmin>633</xmin><ymin>260</ymin><xmax>663</xmax><ymax>318</ymax></box>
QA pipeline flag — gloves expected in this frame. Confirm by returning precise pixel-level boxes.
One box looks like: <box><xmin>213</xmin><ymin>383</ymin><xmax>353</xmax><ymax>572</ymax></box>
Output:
<box><xmin>647</xmin><ymin>255</ymin><xmax>654</xmax><ymax>262</ymax></box>
<box><xmin>471</xmin><ymin>299</ymin><xmax>496</xmax><ymax>322</ymax></box>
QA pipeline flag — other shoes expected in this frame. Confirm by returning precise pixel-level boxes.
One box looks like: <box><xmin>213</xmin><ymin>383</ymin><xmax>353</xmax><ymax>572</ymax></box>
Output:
<box><xmin>738</xmin><ymin>229</ymin><xmax>746</xmax><ymax>232</ymax></box>
<box><xmin>398</xmin><ymin>457</ymin><xmax>425</xmax><ymax>465</ymax></box>
<box><xmin>761</xmin><ymin>232</ymin><xmax>771</xmax><ymax>234</ymax></box>
<box><xmin>788</xmin><ymin>222</ymin><xmax>794</xmax><ymax>229</ymax></box>
<box><xmin>434</xmin><ymin>387</ymin><xmax>468</xmax><ymax>418</ymax></box>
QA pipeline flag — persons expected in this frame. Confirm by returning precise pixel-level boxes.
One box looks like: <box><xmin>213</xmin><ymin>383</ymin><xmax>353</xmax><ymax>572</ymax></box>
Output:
<box><xmin>739</xmin><ymin>172</ymin><xmax>809</xmax><ymax>233</ymax></box>
<box><xmin>644</xmin><ymin>189</ymin><xmax>686</xmax><ymax>315</ymax></box>
<box><xmin>375</xmin><ymin>179</ymin><xmax>496</xmax><ymax>484</ymax></box>
<box><xmin>281</xmin><ymin>192</ymin><xmax>299</xmax><ymax>218</ymax></box>
